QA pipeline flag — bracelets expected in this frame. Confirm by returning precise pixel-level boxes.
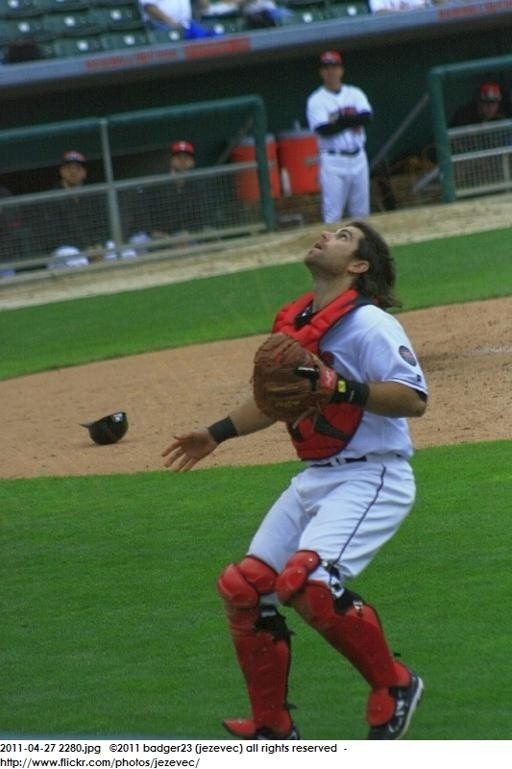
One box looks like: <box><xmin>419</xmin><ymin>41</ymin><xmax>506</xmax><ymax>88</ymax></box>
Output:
<box><xmin>206</xmin><ymin>416</ymin><xmax>239</xmax><ymax>447</ymax></box>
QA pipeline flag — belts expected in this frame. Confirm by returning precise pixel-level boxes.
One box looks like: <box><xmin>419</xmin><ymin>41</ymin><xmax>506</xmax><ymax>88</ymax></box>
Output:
<box><xmin>312</xmin><ymin>457</ymin><xmax>367</xmax><ymax>467</ymax></box>
<box><xmin>328</xmin><ymin>148</ymin><xmax>360</xmax><ymax>156</ymax></box>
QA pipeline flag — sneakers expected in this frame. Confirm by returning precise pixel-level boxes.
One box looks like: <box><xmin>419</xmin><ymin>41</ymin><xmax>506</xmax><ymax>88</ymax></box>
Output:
<box><xmin>368</xmin><ymin>671</ymin><xmax>424</xmax><ymax>740</ymax></box>
<box><xmin>256</xmin><ymin>726</ymin><xmax>300</xmax><ymax>739</ymax></box>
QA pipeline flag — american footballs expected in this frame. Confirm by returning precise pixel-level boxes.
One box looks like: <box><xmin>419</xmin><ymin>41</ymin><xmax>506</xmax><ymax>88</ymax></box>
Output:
<box><xmin>254</xmin><ymin>331</ymin><xmax>337</xmax><ymax>422</ymax></box>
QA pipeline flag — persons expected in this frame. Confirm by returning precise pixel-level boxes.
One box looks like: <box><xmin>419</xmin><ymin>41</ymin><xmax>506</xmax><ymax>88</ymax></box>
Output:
<box><xmin>305</xmin><ymin>49</ymin><xmax>376</xmax><ymax>222</ymax></box>
<box><xmin>369</xmin><ymin>0</ymin><xmax>434</xmax><ymax>18</ymax></box>
<box><xmin>195</xmin><ymin>0</ymin><xmax>242</xmax><ymax>24</ymax></box>
<box><xmin>239</xmin><ymin>2</ymin><xmax>297</xmax><ymax>29</ymax></box>
<box><xmin>35</xmin><ymin>150</ymin><xmax>137</xmax><ymax>272</ymax></box>
<box><xmin>136</xmin><ymin>0</ymin><xmax>213</xmax><ymax>43</ymax></box>
<box><xmin>126</xmin><ymin>138</ymin><xmax>226</xmax><ymax>255</ymax></box>
<box><xmin>159</xmin><ymin>218</ymin><xmax>430</xmax><ymax>741</ymax></box>
<box><xmin>0</xmin><ymin>187</ymin><xmax>28</xmax><ymax>281</ymax></box>
<box><xmin>448</xmin><ymin>82</ymin><xmax>512</xmax><ymax>198</ymax></box>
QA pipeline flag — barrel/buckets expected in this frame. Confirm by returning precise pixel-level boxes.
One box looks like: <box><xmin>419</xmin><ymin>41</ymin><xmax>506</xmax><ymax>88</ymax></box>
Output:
<box><xmin>232</xmin><ymin>133</ymin><xmax>280</xmax><ymax>204</ymax></box>
<box><xmin>278</xmin><ymin>127</ymin><xmax>320</xmax><ymax>195</ymax></box>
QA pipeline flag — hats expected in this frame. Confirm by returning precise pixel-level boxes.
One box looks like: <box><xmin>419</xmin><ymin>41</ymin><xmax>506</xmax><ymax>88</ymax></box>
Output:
<box><xmin>171</xmin><ymin>140</ymin><xmax>195</xmax><ymax>156</ymax></box>
<box><xmin>61</xmin><ymin>152</ymin><xmax>87</xmax><ymax>167</ymax></box>
<box><xmin>320</xmin><ymin>51</ymin><xmax>343</xmax><ymax>65</ymax></box>
<box><xmin>478</xmin><ymin>83</ymin><xmax>502</xmax><ymax>103</ymax></box>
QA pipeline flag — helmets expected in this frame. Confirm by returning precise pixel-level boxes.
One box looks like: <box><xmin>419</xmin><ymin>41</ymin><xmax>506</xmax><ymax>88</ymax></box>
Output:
<box><xmin>79</xmin><ymin>412</ymin><xmax>128</xmax><ymax>444</ymax></box>
